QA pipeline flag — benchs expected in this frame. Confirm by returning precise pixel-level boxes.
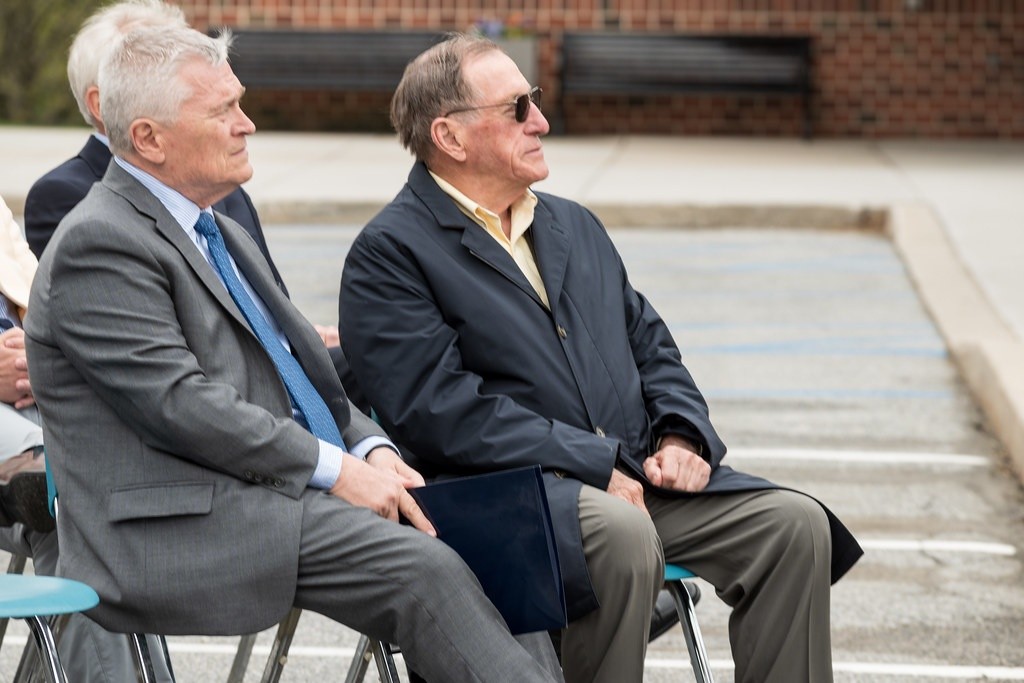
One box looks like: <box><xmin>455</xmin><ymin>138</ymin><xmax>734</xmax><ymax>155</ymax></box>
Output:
<box><xmin>203</xmin><ymin>26</ymin><xmax>454</xmax><ymax>88</ymax></box>
<box><xmin>553</xmin><ymin>29</ymin><xmax>816</xmax><ymax>137</ymax></box>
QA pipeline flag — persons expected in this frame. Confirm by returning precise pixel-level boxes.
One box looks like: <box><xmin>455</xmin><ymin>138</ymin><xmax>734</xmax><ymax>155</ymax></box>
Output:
<box><xmin>0</xmin><ymin>198</ymin><xmax>178</xmax><ymax>683</ymax></box>
<box><xmin>338</xmin><ymin>35</ymin><xmax>865</xmax><ymax>683</ymax></box>
<box><xmin>25</xmin><ymin>0</ymin><xmax>340</xmax><ymax>360</ymax></box>
<box><xmin>19</xmin><ymin>23</ymin><xmax>578</xmax><ymax>683</ymax></box>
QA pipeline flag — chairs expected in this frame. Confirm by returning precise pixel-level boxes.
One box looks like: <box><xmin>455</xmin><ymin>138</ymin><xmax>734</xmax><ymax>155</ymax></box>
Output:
<box><xmin>25</xmin><ymin>437</ymin><xmax>402</xmax><ymax>683</ymax></box>
<box><xmin>343</xmin><ymin>408</ymin><xmax>714</xmax><ymax>683</ymax></box>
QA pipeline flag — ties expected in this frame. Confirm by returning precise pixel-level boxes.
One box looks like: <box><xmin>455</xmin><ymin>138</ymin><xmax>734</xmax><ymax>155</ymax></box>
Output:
<box><xmin>193</xmin><ymin>212</ymin><xmax>349</xmax><ymax>453</ymax></box>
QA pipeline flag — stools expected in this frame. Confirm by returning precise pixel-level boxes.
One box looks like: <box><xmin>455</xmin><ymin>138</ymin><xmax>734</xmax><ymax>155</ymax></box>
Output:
<box><xmin>0</xmin><ymin>573</ymin><xmax>100</xmax><ymax>683</ymax></box>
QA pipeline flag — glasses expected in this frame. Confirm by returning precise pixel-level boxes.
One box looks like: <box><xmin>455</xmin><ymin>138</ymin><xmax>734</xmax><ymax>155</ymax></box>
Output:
<box><xmin>444</xmin><ymin>86</ymin><xmax>543</xmax><ymax>123</ymax></box>
